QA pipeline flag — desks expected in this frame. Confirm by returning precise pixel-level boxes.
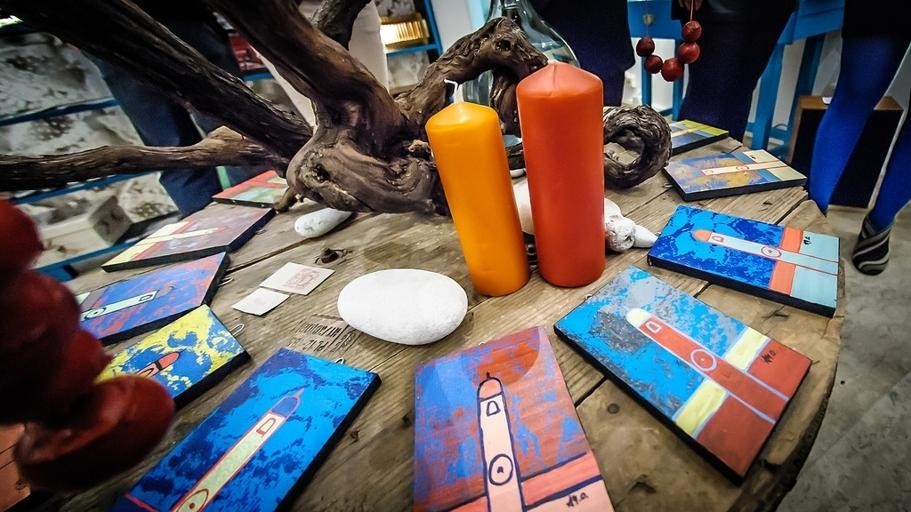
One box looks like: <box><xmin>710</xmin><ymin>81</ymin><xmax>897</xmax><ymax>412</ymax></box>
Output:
<box><xmin>28</xmin><ymin>108</ymin><xmax>849</xmax><ymax>512</ymax></box>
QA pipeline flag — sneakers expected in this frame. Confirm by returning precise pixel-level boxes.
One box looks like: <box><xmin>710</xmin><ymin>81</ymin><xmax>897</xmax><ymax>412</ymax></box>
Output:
<box><xmin>852</xmin><ymin>211</ymin><xmax>896</xmax><ymax>275</ymax></box>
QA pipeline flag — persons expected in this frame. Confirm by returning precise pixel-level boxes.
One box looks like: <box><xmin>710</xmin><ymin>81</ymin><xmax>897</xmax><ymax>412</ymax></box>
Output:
<box><xmin>527</xmin><ymin>1</ymin><xmax>637</xmax><ymax>109</ymax></box>
<box><xmin>809</xmin><ymin>1</ymin><xmax>911</xmax><ymax>275</ymax></box>
<box><xmin>68</xmin><ymin>1</ymin><xmax>268</xmax><ymax>218</ymax></box>
<box><xmin>670</xmin><ymin>2</ymin><xmax>804</xmax><ymax>144</ymax></box>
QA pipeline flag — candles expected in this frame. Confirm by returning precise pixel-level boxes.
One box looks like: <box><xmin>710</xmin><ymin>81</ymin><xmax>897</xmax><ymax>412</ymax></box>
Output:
<box><xmin>423</xmin><ymin>77</ymin><xmax>532</xmax><ymax>299</ymax></box>
<box><xmin>515</xmin><ymin>58</ymin><xmax>607</xmax><ymax>290</ymax></box>
